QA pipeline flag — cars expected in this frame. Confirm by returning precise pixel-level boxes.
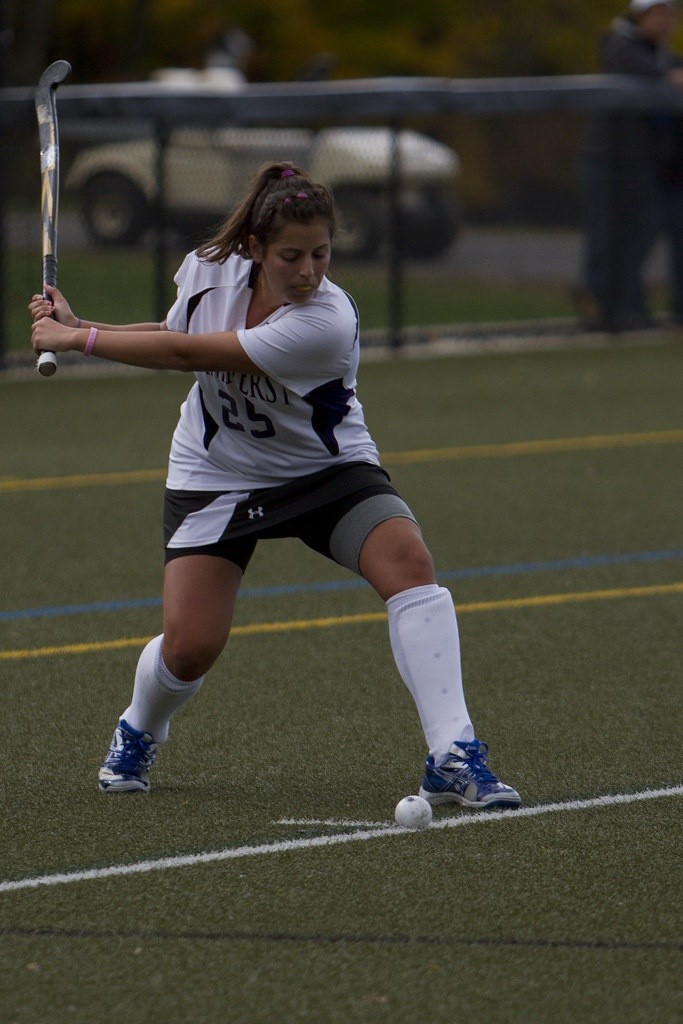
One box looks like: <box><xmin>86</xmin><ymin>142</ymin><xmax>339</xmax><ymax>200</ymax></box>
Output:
<box><xmin>67</xmin><ymin>114</ymin><xmax>470</xmax><ymax>268</ymax></box>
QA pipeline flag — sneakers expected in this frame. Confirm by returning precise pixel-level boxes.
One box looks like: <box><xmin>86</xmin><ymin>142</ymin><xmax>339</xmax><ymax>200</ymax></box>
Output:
<box><xmin>419</xmin><ymin>738</ymin><xmax>522</xmax><ymax>810</ymax></box>
<box><xmin>98</xmin><ymin>705</ymin><xmax>160</xmax><ymax>793</ymax></box>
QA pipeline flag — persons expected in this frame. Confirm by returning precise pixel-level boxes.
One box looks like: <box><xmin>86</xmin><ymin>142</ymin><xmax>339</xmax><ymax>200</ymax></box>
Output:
<box><xmin>572</xmin><ymin>0</ymin><xmax>682</xmax><ymax>333</ymax></box>
<box><xmin>28</xmin><ymin>161</ymin><xmax>522</xmax><ymax>810</ymax></box>
<box><xmin>206</xmin><ymin>29</ymin><xmax>251</xmax><ymax>67</ymax></box>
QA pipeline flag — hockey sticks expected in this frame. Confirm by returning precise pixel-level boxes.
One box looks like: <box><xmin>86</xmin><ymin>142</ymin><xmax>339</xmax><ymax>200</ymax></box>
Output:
<box><xmin>34</xmin><ymin>59</ymin><xmax>72</xmax><ymax>375</ymax></box>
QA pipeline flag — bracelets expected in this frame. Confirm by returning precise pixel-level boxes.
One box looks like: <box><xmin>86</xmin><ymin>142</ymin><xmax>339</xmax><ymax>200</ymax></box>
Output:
<box><xmin>77</xmin><ymin>319</ymin><xmax>81</xmax><ymax>327</ymax></box>
<box><xmin>83</xmin><ymin>327</ymin><xmax>98</xmax><ymax>358</ymax></box>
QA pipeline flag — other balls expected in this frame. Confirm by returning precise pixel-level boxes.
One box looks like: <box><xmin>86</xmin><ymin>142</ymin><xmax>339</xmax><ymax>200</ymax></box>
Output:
<box><xmin>395</xmin><ymin>794</ymin><xmax>433</xmax><ymax>832</ymax></box>
